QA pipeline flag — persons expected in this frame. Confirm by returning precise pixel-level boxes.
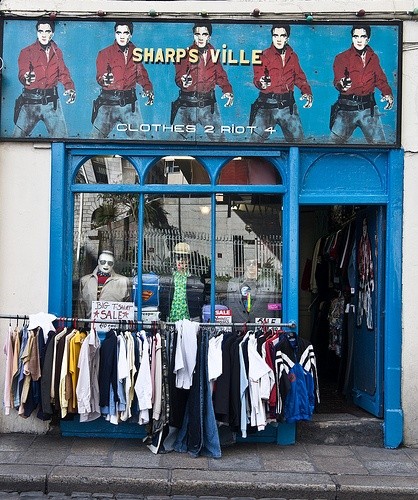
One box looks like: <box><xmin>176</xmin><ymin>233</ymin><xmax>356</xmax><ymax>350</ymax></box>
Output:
<box><xmin>227</xmin><ymin>247</ymin><xmax>278</xmax><ymax>329</ymax></box>
<box><xmin>157</xmin><ymin>243</ymin><xmax>205</xmax><ymax>323</ymax></box>
<box><xmin>78</xmin><ymin>242</ymin><xmax>132</xmax><ymax>320</ymax></box>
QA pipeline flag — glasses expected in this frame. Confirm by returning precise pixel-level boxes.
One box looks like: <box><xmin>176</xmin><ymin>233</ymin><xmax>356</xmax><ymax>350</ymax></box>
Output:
<box><xmin>99</xmin><ymin>260</ymin><xmax>114</xmax><ymax>266</ymax></box>
<box><xmin>177</xmin><ymin>255</ymin><xmax>186</xmax><ymax>260</ymax></box>
<box><xmin>246</xmin><ymin>261</ymin><xmax>256</xmax><ymax>266</ymax></box>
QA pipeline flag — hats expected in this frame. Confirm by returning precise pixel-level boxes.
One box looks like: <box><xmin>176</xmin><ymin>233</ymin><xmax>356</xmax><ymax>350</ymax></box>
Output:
<box><xmin>172</xmin><ymin>242</ymin><xmax>191</xmax><ymax>254</ymax></box>
<box><xmin>244</xmin><ymin>252</ymin><xmax>257</xmax><ymax>260</ymax></box>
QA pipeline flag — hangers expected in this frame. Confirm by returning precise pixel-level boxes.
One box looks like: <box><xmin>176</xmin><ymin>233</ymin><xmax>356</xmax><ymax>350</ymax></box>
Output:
<box><xmin>9</xmin><ymin>315</ymin><xmax>286</xmax><ymax>339</ymax></box>
